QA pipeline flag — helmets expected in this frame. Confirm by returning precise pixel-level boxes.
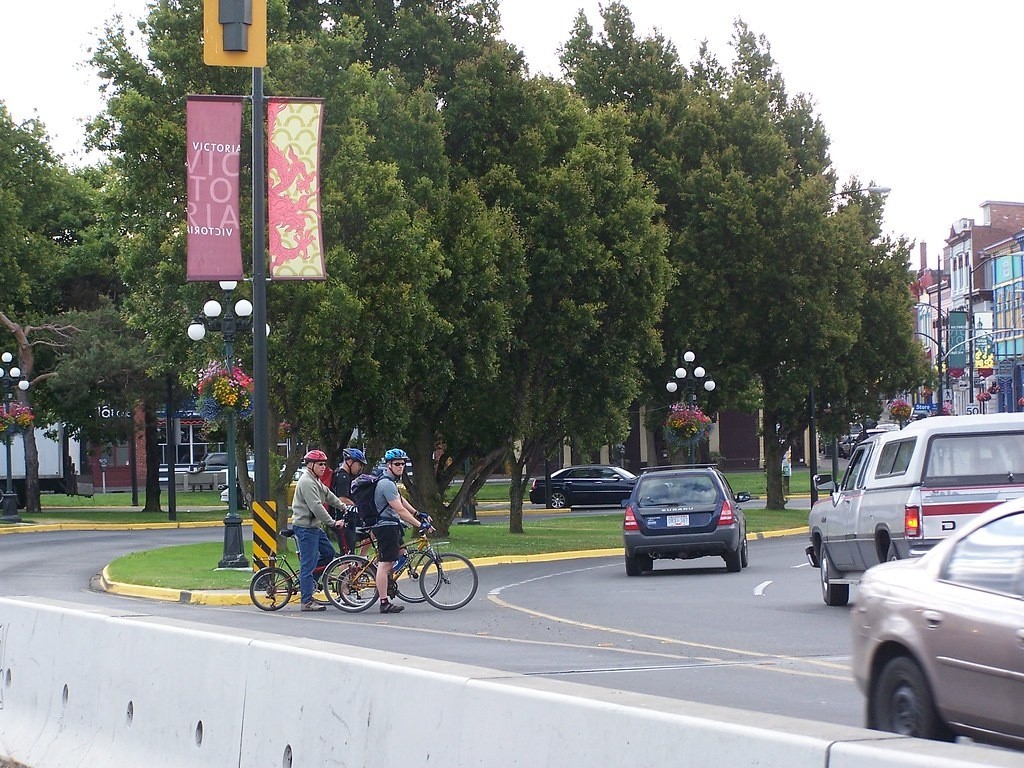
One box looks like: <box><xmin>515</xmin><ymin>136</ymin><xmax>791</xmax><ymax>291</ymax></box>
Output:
<box><xmin>343</xmin><ymin>448</ymin><xmax>367</xmax><ymax>466</ymax></box>
<box><xmin>385</xmin><ymin>449</ymin><xmax>408</xmax><ymax>460</ymax></box>
<box><xmin>304</xmin><ymin>450</ymin><xmax>328</xmax><ymax>461</ymax></box>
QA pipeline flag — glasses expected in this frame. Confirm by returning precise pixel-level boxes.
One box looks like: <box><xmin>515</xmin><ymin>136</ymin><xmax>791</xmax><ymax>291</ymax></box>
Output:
<box><xmin>394</xmin><ymin>463</ymin><xmax>406</xmax><ymax>466</ymax></box>
<box><xmin>319</xmin><ymin>463</ymin><xmax>327</xmax><ymax>466</ymax></box>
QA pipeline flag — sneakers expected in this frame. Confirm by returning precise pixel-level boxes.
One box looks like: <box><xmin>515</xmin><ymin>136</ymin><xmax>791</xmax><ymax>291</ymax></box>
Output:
<box><xmin>380</xmin><ymin>602</ymin><xmax>405</xmax><ymax>613</ymax></box>
<box><xmin>339</xmin><ymin>595</ymin><xmax>367</xmax><ymax>605</ymax></box>
<box><xmin>300</xmin><ymin>600</ymin><xmax>327</xmax><ymax>611</ymax></box>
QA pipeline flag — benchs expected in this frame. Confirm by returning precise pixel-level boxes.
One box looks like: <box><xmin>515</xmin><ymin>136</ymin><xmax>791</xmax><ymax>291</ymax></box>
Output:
<box><xmin>174</xmin><ymin>471</ymin><xmax>227</xmax><ymax>492</ymax></box>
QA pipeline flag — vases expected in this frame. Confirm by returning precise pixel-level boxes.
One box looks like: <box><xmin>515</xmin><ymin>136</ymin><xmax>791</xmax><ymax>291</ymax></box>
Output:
<box><xmin>894</xmin><ymin>412</ymin><xmax>910</xmax><ymax>420</ymax></box>
<box><xmin>0</xmin><ymin>430</ymin><xmax>11</xmax><ymax>442</ymax></box>
<box><xmin>242</xmin><ymin>429</ymin><xmax>255</xmax><ymax>443</ymax></box>
<box><xmin>206</xmin><ymin>430</ymin><xmax>225</xmax><ymax>445</ymax></box>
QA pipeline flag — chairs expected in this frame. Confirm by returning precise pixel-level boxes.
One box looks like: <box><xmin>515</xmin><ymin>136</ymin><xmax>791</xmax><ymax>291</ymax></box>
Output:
<box><xmin>643</xmin><ymin>481</ymin><xmax>673</xmax><ymax>504</ymax></box>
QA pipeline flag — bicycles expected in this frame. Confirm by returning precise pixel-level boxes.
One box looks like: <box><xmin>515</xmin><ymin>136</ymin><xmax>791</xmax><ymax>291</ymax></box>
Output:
<box><xmin>249</xmin><ymin>513</ymin><xmax>479</xmax><ymax>613</ymax></box>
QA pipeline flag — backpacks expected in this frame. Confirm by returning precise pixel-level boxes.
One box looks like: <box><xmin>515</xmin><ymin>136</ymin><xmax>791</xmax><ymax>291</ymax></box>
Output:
<box><xmin>350</xmin><ymin>474</ymin><xmax>397</xmax><ymax>526</ymax></box>
<box><xmin>321</xmin><ymin>467</ymin><xmax>346</xmax><ymax>514</ymax></box>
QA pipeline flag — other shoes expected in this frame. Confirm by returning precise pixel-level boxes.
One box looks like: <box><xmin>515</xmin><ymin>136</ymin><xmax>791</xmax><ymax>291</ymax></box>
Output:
<box><xmin>312</xmin><ymin>582</ymin><xmax>324</xmax><ymax>592</ymax></box>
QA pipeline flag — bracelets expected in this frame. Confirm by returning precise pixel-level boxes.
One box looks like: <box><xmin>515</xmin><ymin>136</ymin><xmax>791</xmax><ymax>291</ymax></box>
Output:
<box><xmin>413</xmin><ymin>510</ymin><xmax>419</xmax><ymax>518</ymax></box>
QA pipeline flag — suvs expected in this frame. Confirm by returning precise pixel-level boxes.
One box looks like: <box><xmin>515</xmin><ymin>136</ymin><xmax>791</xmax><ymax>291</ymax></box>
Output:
<box><xmin>622</xmin><ymin>463</ymin><xmax>752</xmax><ymax>576</ymax></box>
<box><xmin>803</xmin><ymin>416</ymin><xmax>1023</xmax><ymax>606</ymax></box>
<box><xmin>200</xmin><ymin>453</ymin><xmax>230</xmax><ymax>471</ymax></box>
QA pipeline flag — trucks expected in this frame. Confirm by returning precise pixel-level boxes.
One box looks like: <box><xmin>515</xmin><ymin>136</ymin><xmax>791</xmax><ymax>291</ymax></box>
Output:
<box><xmin>0</xmin><ymin>414</ymin><xmax>81</xmax><ymax>497</ymax></box>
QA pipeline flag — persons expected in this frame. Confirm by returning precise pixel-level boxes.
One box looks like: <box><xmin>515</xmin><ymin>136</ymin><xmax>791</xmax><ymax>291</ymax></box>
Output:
<box><xmin>329</xmin><ymin>448</ymin><xmax>376</xmax><ymax>606</ymax></box>
<box><xmin>372</xmin><ymin>449</ymin><xmax>434</xmax><ymax>613</ymax></box>
<box><xmin>292</xmin><ymin>450</ymin><xmax>351</xmax><ymax>611</ymax></box>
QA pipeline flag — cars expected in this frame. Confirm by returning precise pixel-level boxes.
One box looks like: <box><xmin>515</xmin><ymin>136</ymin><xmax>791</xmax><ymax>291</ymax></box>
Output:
<box><xmin>528</xmin><ymin>463</ymin><xmax>638</xmax><ymax>508</ymax></box>
<box><xmin>817</xmin><ymin>411</ymin><xmax>890</xmax><ymax>461</ymax></box>
<box><xmin>235</xmin><ymin>460</ymin><xmax>255</xmax><ymax>482</ymax></box>
<box><xmin>851</xmin><ymin>496</ymin><xmax>1023</xmax><ymax>754</ymax></box>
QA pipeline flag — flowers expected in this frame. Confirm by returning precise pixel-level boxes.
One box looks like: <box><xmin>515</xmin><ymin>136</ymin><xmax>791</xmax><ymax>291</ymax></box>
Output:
<box><xmin>665</xmin><ymin>403</ymin><xmax>712</xmax><ymax>448</ymax></box>
<box><xmin>197</xmin><ymin>363</ymin><xmax>252</xmax><ymax>424</ymax></box>
<box><xmin>890</xmin><ymin>400</ymin><xmax>912</xmax><ymax>415</ymax></box>
<box><xmin>197</xmin><ymin>410</ymin><xmax>231</xmax><ymax>436</ymax></box>
<box><xmin>0</xmin><ymin>403</ymin><xmax>35</xmax><ymax>432</ymax></box>
<box><xmin>275</xmin><ymin>419</ymin><xmax>292</xmax><ymax>441</ymax></box>
<box><xmin>236</xmin><ymin>418</ymin><xmax>259</xmax><ymax>432</ymax></box>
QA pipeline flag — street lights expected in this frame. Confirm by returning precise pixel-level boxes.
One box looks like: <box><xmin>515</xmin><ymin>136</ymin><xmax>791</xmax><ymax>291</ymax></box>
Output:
<box><xmin>187</xmin><ymin>281</ymin><xmax>271</xmax><ymax>569</ymax></box>
<box><xmin>966</xmin><ymin>247</ymin><xmax>1024</xmax><ymax>414</ymax></box>
<box><xmin>666</xmin><ymin>350</ymin><xmax>717</xmax><ymax>467</ymax></box>
<box><xmin>0</xmin><ymin>351</ymin><xmax>30</xmax><ymax>524</ymax></box>
<box><xmin>807</xmin><ymin>186</ymin><xmax>892</xmax><ymax>505</ymax></box>
